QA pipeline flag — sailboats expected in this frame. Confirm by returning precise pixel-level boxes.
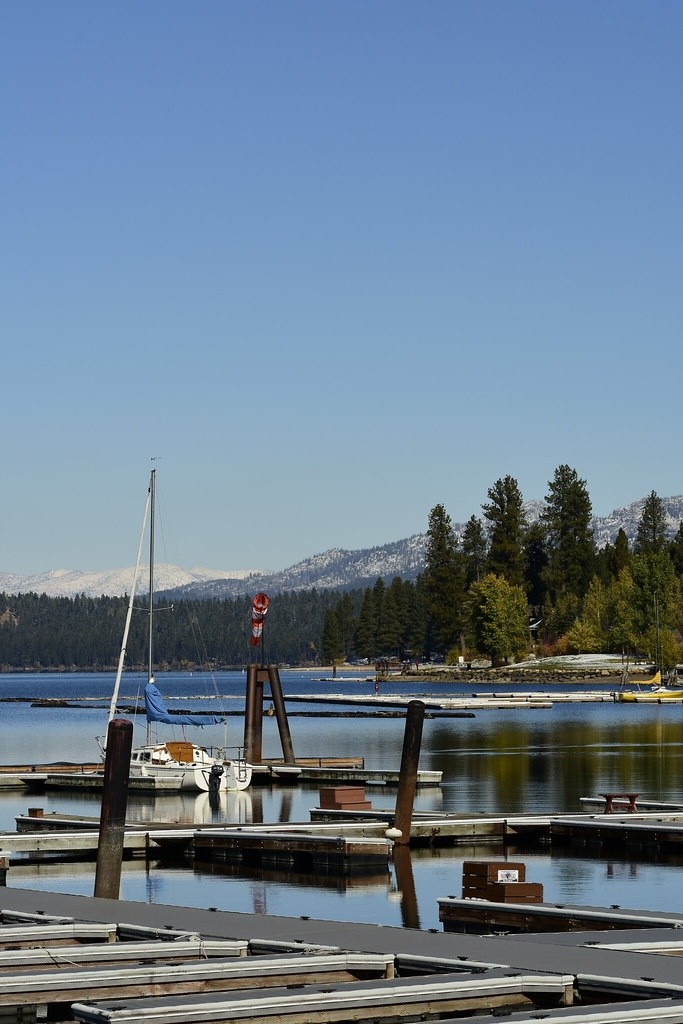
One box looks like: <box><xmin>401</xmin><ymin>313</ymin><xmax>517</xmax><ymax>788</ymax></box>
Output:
<box><xmin>94</xmin><ymin>456</ymin><xmax>253</xmax><ymax>793</ymax></box>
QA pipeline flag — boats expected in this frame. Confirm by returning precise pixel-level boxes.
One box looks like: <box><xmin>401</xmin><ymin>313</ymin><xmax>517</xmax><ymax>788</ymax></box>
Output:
<box><xmin>629</xmin><ymin>670</ymin><xmax>663</xmax><ymax>685</ymax></box>
<box><xmin>618</xmin><ymin>688</ymin><xmax>683</xmax><ymax>703</ymax></box>
